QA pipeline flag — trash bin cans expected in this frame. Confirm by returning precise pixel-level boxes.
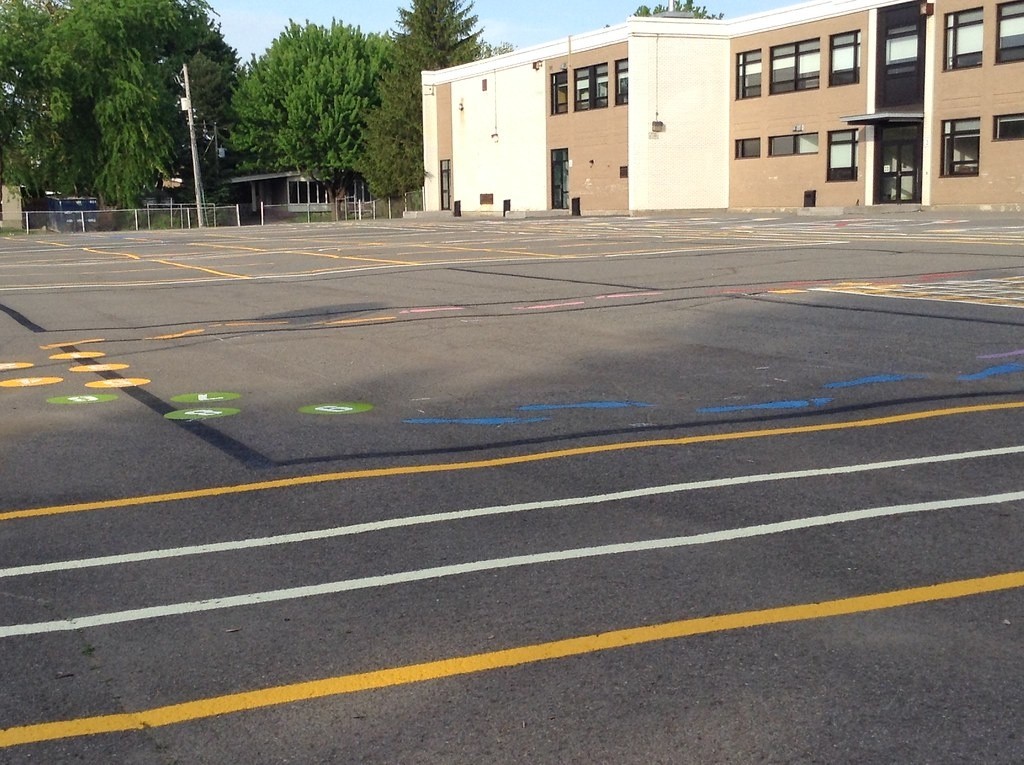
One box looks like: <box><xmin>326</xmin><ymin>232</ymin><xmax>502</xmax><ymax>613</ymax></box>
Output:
<box><xmin>804</xmin><ymin>190</ymin><xmax>817</xmax><ymax>207</ymax></box>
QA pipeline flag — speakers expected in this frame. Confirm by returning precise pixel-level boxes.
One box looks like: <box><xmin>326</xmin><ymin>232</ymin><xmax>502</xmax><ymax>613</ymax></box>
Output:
<box><xmin>181</xmin><ymin>98</ymin><xmax>189</xmax><ymax>111</ymax></box>
